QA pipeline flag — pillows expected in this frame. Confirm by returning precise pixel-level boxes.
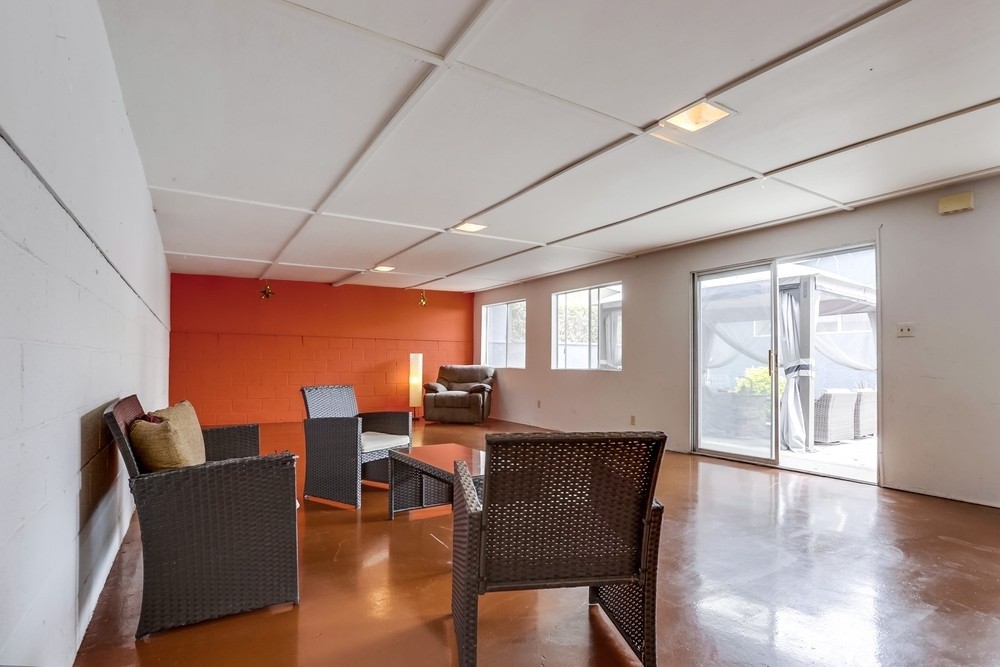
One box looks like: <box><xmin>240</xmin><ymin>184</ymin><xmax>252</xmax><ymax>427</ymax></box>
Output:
<box><xmin>129</xmin><ymin>400</ymin><xmax>206</xmax><ymax>472</ymax></box>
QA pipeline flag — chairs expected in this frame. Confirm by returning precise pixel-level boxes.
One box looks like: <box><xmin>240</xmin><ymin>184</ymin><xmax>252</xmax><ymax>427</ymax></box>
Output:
<box><xmin>814</xmin><ymin>389</ymin><xmax>877</xmax><ymax>443</ymax></box>
<box><xmin>300</xmin><ymin>384</ymin><xmax>412</xmax><ymax>509</ymax></box>
<box><xmin>104</xmin><ymin>394</ymin><xmax>298</xmax><ymax>641</ymax></box>
<box><xmin>452</xmin><ymin>430</ymin><xmax>668</xmax><ymax>667</ymax></box>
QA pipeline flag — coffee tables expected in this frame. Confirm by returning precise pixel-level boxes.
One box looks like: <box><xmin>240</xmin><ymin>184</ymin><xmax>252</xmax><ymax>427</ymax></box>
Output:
<box><xmin>388</xmin><ymin>444</ymin><xmax>486</xmax><ymax>521</ymax></box>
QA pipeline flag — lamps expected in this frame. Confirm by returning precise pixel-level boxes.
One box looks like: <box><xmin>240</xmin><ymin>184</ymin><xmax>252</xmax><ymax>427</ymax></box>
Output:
<box><xmin>409</xmin><ymin>353</ymin><xmax>423</xmax><ymax>421</ymax></box>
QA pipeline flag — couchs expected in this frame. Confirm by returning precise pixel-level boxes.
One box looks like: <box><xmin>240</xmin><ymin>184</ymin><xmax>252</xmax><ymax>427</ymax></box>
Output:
<box><xmin>423</xmin><ymin>364</ymin><xmax>496</xmax><ymax>423</ymax></box>
